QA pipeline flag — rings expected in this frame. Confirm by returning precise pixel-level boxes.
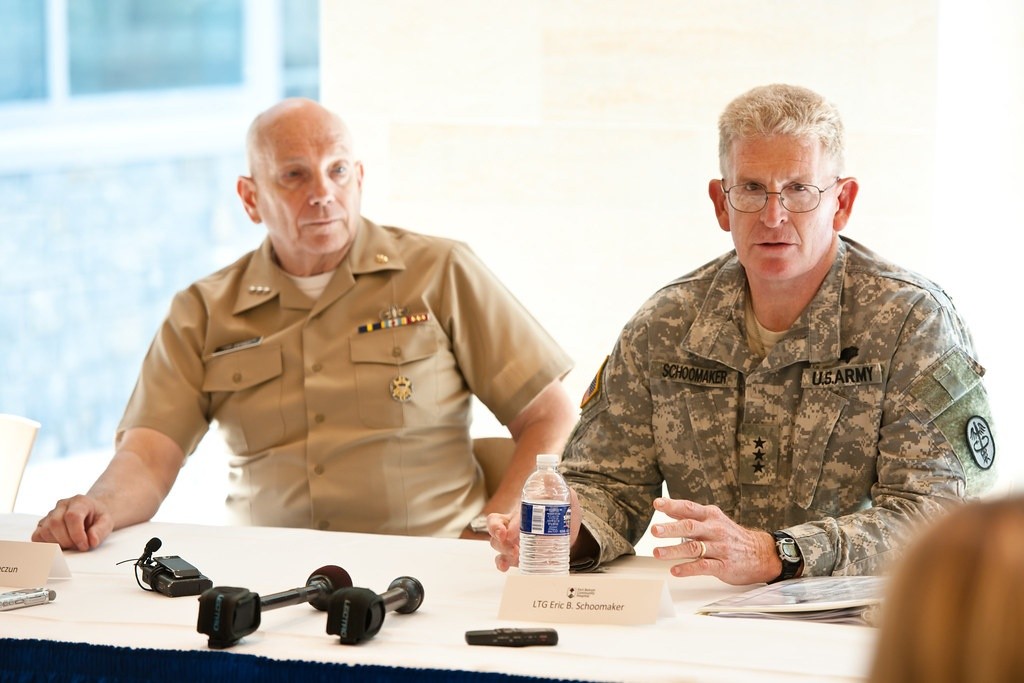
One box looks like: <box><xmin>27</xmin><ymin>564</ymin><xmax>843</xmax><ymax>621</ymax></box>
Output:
<box><xmin>699</xmin><ymin>541</ymin><xmax>706</xmax><ymax>558</ymax></box>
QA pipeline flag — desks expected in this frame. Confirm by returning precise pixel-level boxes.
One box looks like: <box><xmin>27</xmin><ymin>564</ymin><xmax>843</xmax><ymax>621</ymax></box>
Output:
<box><xmin>0</xmin><ymin>515</ymin><xmax>877</xmax><ymax>683</ymax></box>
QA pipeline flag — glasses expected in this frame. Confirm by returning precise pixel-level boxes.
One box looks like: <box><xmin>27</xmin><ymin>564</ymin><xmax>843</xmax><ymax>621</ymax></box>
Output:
<box><xmin>721</xmin><ymin>178</ymin><xmax>839</xmax><ymax>213</ymax></box>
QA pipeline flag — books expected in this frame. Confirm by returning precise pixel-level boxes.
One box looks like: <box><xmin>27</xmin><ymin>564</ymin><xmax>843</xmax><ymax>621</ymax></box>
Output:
<box><xmin>695</xmin><ymin>576</ymin><xmax>887</xmax><ymax>627</ymax></box>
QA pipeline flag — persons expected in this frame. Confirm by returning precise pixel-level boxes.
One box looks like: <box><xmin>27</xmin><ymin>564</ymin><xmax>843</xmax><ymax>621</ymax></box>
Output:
<box><xmin>31</xmin><ymin>99</ymin><xmax>578</xmax><ymax>551</ymax></box>
<box><xmin>487</xmin><ymin>83</ymin><xmax>997</xmax><ymax>586</ymax></box>
<box><xmin>868</xmin><ymin>494</ymin><xmax>1024</xmax><ymax>683</ymax></box>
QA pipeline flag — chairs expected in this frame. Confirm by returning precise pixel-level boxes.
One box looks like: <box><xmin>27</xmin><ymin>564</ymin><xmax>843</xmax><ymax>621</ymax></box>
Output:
<box><xmin>0</xmin><ymin>413</ymin><xmax>41</xmax><ymax>512</ymax></box>
<box><xmin>459</xmin><ymin>438</ymin><xmax>517</xmax><ymax>540</ymax></box>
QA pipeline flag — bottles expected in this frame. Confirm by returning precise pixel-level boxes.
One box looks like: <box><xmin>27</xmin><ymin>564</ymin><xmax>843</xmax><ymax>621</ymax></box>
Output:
<box><xmin>519</xmin><ymin>454</ymin><xmax>572</xmax><ymax>573</ymax></box>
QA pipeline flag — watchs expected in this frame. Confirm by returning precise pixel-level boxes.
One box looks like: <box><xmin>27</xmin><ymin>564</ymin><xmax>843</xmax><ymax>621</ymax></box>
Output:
<box><xmin>766</xmin><ymin>532</ymin><xmax>803</xmax><ymax>585</ymax></box>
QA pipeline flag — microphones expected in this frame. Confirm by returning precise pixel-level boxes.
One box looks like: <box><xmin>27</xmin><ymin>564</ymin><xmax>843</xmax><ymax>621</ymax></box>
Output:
<box><xmin>138</xmin><ymin>538</ymin><xmax>162</xmax><ymax>566</ymax></box>
<box><xmin>196</xmin><ymin>565</ymin><xmax>353</xmax><ymax>650</ymax></box>
<box><xmin>326</xmin><ymin>577</ymin><xmax>425</xmax><ymax>645</ymax></box>
